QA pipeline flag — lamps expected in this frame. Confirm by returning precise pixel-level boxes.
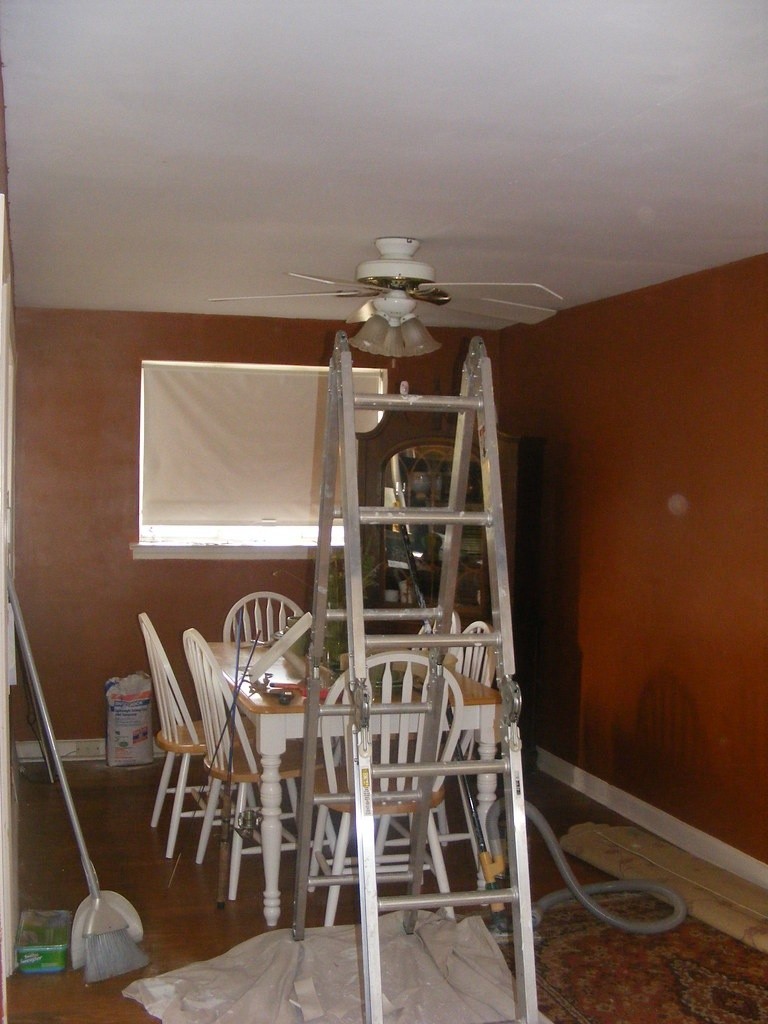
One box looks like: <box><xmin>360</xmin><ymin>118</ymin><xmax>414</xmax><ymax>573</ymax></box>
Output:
<box><xmin>347</xmin><ymin>238</ymin><xmax>442</xmax><ymax>357</ymax></box>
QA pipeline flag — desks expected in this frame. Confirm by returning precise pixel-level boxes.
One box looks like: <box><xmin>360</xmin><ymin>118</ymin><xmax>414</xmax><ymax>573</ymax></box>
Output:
<box><xmin>209</xmin><ymin>639</ymin><xmax>502</xmax><ymax>929</ymax></box>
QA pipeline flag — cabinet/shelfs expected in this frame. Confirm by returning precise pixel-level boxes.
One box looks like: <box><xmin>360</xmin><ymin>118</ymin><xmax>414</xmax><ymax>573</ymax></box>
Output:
<box><xmin>355</xmin><ymin>410</ymin><xmax>545</xmax><ymax>775</ymax></box>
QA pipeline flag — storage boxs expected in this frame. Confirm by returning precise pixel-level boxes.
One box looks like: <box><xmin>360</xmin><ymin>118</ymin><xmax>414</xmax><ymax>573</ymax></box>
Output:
<box><xmin>15</xmin><ymin>909</ymin><xmax>72</xmax><ymax>973</ymax></box>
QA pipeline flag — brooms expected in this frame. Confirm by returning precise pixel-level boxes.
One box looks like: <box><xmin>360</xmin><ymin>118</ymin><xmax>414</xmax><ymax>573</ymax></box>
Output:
<box><xmin>5</xmin><ymin>569</ymin><xmax>152</xmax><ymax>984</ymax></box>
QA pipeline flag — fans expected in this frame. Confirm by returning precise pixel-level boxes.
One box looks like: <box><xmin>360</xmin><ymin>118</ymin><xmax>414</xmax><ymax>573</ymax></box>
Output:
<box><xmin>208</xmin><ymin>238</ymin><xmax>565</xmax><ymax>325</ymax></box>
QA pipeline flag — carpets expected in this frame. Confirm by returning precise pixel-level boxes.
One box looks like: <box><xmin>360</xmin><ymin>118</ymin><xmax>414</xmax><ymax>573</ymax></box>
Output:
<box><xmin>454</xmin><ymin>892</ymin><xmax>768</xmax><ymax>1024</ymax></box>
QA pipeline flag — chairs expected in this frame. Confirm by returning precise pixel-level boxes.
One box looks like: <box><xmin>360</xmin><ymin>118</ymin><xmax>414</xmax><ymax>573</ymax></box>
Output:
<box><xmin>181</xmin><ymin>627</ymin><xmax>326</xmax><ymax>898</ymax></box>
<box><xmin>375</xmin><ymin>619</ymin><xmax>497</xmax><ymax>849</ymax></box>
<box><xmin>366</xmin><ymin>609</ymin><xmax>460</xmax><ymax>753</ymax></box>
<box><xmin>137</xmin><ymin>610</ymin><xmax>257</xmax><ymax>858</ymax></box>
<box><xmin>307</xmin><ymin>651</ymin><xmax>463</xmax><ymax>928</ymax></box>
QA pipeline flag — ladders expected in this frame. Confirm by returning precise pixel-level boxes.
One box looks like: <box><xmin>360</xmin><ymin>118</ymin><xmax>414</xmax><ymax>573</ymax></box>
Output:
<box><xmin>294</xmin><ymin>328</ymin><xmax>542</xmax><ymax>1024</ymax></box>
